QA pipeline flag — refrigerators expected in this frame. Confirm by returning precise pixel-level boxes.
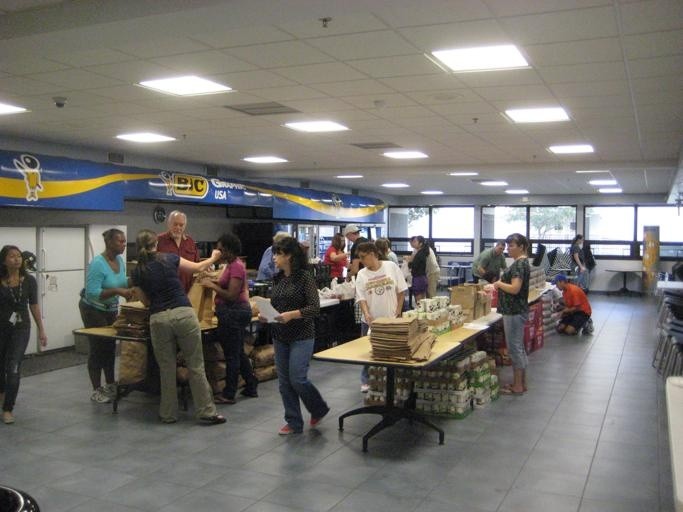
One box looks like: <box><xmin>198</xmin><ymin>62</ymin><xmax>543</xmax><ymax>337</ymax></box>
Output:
<box><xmin>235</xmin><ymin>225</ymin><xmax>386</xmax><ymax>270</ymax></box>
<box><xmin>0</xmin><ymin>223</ymin><xmax>127</xmax><ymax>362</ymax></box>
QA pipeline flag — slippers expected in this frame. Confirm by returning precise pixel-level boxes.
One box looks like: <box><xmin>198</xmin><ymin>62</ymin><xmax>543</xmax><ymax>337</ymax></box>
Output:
<box><xmin>214</xmin><ymin>395</ymin><xmax>237</xmax><ymax>405</ymax></box>
<box><xmin>499</xmin><ymin>382</ymin><xmax>528</xmax><ymax>396</ymax></box>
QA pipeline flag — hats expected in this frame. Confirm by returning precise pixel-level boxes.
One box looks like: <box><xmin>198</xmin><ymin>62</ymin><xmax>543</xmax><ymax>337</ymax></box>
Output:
<box><xmin>343</xmin><ymin>224</ymin><xmax>361</xmax><ymax>235</ymax></box>
<box><xmin>550</xmin><ymin>274</ymin><xmax>567</xmax><ymax>285</ymax></box>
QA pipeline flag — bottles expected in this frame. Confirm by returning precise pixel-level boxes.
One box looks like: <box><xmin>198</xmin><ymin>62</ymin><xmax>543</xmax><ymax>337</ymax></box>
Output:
<box><xmin>664</xmin><ymin>271</ymin><xmax>668</xmax><ymax>284</ymax></box>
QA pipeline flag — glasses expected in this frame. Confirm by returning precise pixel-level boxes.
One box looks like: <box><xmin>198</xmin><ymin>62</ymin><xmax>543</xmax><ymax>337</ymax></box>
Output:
<box><xmin>358</xmin><ymin>252</ymin><xmax>369</xmax><ymax>259</ymax></box>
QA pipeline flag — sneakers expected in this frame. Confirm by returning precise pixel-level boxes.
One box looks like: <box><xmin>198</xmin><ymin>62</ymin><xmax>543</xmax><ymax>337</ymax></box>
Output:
<box><xmin>309</xmin><ymin>408</ymin><xmax>331</xmax><ymax>429</ymax></box>
<box><xmin>239</xmin><ymin>390</ymin><xmax>259</xmax><ymax>398</ymax></box>
<box><xmin>104</xmin><ymin>382</ymin><xmax>118</xmax><ymax>394</ymax></box>
<box><xmin>91</xmin><ymin>387</ymin><xmax>112</xmax><ymax>404</ymax></box>
<box><xmin>581</xmin><ymin>318</ymin><xmax>594</xmax><ymax>335</ymax></box>
<box><xmin>279</xmin><ymin>423</ymin><xmax>298</xmax><ymax>436</ymax></box>
<box><xmin>0</xmin><ymin>398</ymin><xmax>16</xmax><ymax>424</ymax></box>
<box><xmin>360</xmin><ymin>382</ymin><xmax>369</xmax><ymax>394</ymax></box>
<box><xmin>199</xmin><ymin>415</ymin><xmax>227</xmax><ymax>425</ymax></box>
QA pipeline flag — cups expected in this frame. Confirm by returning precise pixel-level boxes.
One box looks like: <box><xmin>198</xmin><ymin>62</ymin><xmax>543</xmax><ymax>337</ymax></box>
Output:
<box><xmin>365</xmin><ymin>348</ymin><xmax>500</xmax><ymax>416</ymax></box>
<box><xmin>402</xmin><ymin>296</ymin><xmax>464</xmax><ymax>337</ymax></box>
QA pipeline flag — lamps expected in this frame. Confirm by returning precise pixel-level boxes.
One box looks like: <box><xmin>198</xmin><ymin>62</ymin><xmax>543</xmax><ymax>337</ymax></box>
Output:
<box><xmin>0</xmin><ymin>43</ymin><xmax>626</xmax><ymax>197</ymax></box>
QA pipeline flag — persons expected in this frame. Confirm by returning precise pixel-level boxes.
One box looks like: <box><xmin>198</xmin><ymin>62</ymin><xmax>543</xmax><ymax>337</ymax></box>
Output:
<box><xmin>298</xmin><ymin>222</ymin><xmax>440</xmax><ymax>311</ymax></box>
<box><xmin>255</xmin><ymin>230</ymin><xmax>290</xmax><ymax>295</ymax></box>
<box><xmin>471</xmin><ymin>239</ymin><xmax>507</xmax><ymax>284</ymax></box>
<box><xmin>569</xmin><ymin>233</ymin><xmax>587</xmax><ymax>291</ymax></box>
<box><xmin>0</xmin><ymin>245</ymin><xmax>47</xmax><ymax>425</ymax></box>
<box><xmin>154</xmin><ymin>209</ymin><xmax>203</xmax><ymax>296</ymax></box>
<box><xmin>76</xmin><ymin>228</ymin><xmax>134</xmax><ymax>405</ymax></box>
<box><xmin>128</xmin><ymin>229</ymin><xmax>225</xmax><ymax>425</ymax></box>
<box><xmin>550</xmin><ymin>274</ymin><xmax>594</xmax><ymax>335</ymax></box>
<box><xmin>197</xmin><ymin>230</ymin><xmax>259</xmax><ymax>404</ymax></box>
<box><xmin>257</xmin><ymin>235</ymin><xmax>330</xmax><ymax>435</ymax></box>
<box><xmin>493</xmin><ymin>233</ymin><xmax>531</xmax><ymax>396</ymax></box>
<box><xmin>354</xmin><ymin>242</ymin><xmax>409</xmax><ymax>392</ymax></box>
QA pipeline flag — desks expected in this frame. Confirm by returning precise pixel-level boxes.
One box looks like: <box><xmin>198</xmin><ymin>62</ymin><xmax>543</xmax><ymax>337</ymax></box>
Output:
<box><xmin>312</xmin><ymin>284</ymin><xmax>557</xmax><ymax>454</ymax></box>
<box><xmin>603</xmin><ymin>268</ymin><xmax>645</xmax><ymax>298</ymax></box>
<box><xmin>73</xmin><ymin>296</ymin><xmax>361</xmax><ymax>424</ymax></box>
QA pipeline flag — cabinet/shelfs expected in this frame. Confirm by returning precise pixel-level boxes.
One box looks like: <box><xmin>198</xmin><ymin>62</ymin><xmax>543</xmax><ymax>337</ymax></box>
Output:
<box><xmin>438</xmin><ymin>261</ymin><xmax>475</xmax><ymax>291</ymax></box>
